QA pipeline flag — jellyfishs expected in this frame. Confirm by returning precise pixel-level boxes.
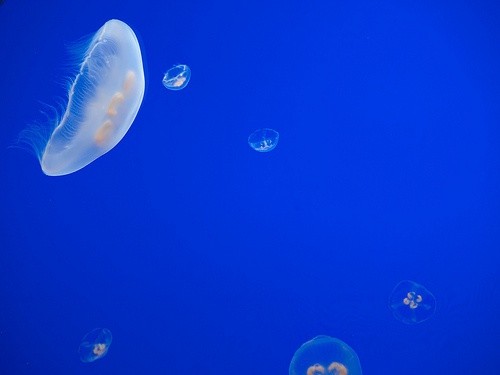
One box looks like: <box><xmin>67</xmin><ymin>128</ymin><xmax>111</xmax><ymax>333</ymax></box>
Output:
<box><xmin>388</xmin><ymin>281</ymin><xmax>438</xmax><ymax>326</ymax></box>
<box><xmin>247</xmin><ymin>129</ymin><xmax>281</xmax><ymax>154</ymax></box>
<box><xmin>18</xmin><ymin>19</ymin><xmax>146</xmax><ymax>178</ymax></box>
<box><xmin>77</xmin><ymin>327</ymin><xmax>114</xmax><ymax>362</ymax></box>
<box><xmin>162</xmin><ymin>65</ymin><xmax>192</xmax><ymax>91</ymax></box>
<box><xmin>288</xmin><ymin>335</ymin><xmax>364</xmax><ymax>375</ymax></box>
